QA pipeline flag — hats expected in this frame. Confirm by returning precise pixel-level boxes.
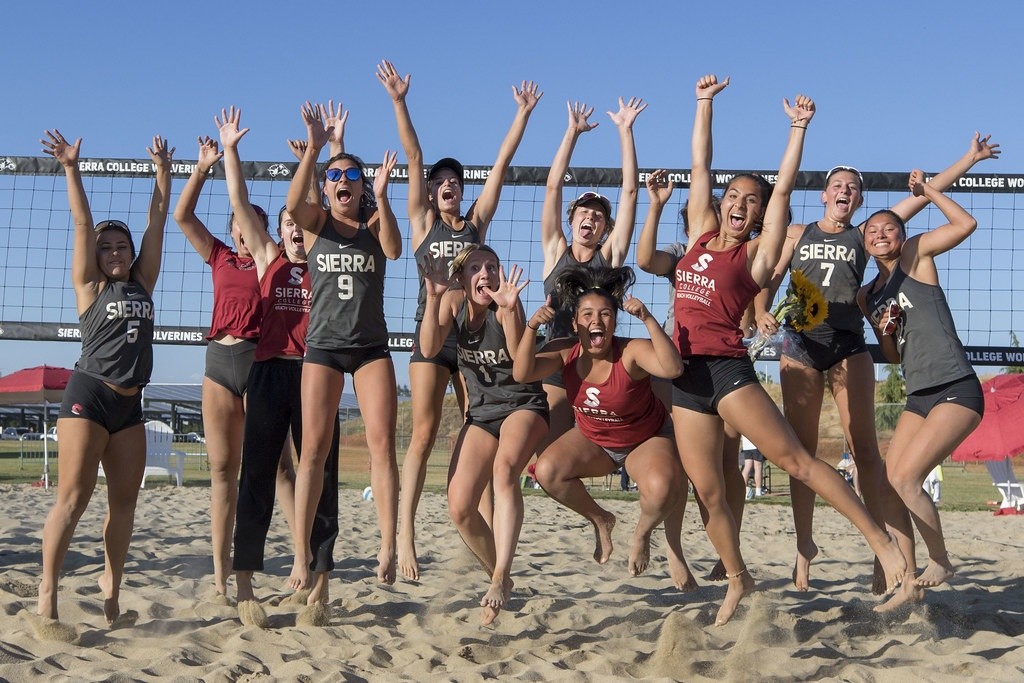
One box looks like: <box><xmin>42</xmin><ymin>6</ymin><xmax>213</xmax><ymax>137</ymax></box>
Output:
<box><xmin>426</xmin><ymin>157</ymin><xmax>464</xmax><ymax>194</ymax></box>
<box><xmin>572</xmin><ymin>197</ymin><xmax>611</xmax><ymax>221</ymax></box>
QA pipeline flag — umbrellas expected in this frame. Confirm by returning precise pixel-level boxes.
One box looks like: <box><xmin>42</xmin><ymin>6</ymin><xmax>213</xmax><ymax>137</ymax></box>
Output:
<box><xmin>0</xmin><ymin>362</ymin><xmax>75</xmax><ymax>490</ymax></box>
<box><xmin>948</xmin><ymin>371</ymin><xmax>1024</xmax><ymax>467</ymax></box>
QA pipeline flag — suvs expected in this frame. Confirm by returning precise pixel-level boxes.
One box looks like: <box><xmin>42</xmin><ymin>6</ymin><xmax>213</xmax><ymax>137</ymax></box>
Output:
<box><xmin>2</xmin><ymin>427</ymin><xmax>35</xmax><ymax>440</ymax></box>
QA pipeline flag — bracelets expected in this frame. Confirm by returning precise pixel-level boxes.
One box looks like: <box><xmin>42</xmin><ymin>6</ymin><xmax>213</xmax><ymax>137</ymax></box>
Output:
<box><xmin>791</xmin><ymin>125</ymin><xmax>807</xmax><ymax>129</ymax></box>
<box><xmin>526</xmin><ymin>321</ymin><xmax>542</xmax><ymax>331</ymax></box>
<box><xmin>790</xmin><ymin>116</ymin><xmax>810</xmax><ymax>124</ymax></box>
<box><xmin>696</xmin><ymin>97</ymin><xmax>714</xmax><ymax>102</ymax></box>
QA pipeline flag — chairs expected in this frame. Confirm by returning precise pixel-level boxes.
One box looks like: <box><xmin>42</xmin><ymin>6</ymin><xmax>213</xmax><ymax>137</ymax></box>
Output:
<box><xmin>986</xmin><ymin>459</ymin><xmax>1024</xmax><ymax>512</ymax></box>
<box><xmin>138</xmin><ymin>420</ymin><xmax>184</xmax><ymax>488</ymax></box>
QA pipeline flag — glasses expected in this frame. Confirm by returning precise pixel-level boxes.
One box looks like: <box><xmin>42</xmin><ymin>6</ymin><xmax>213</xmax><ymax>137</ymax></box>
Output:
<box><xmin>94</xmin><ymin>220</ymin><xmax>132</xmax><ymax>237</ymax></box>
<box><xmin>826</xmin><ymin>166</ymin><xmax>863</xmax><ymax>183</ymax></box>
<box><xmin>577</xmin><ymin>191</ymin><xmax>613</xmax><ymax>209</ymax></box>
<box><xmin>325</xmin><ymin>167</ymin><xmax>362</xmax><ymax>181</ymax></box>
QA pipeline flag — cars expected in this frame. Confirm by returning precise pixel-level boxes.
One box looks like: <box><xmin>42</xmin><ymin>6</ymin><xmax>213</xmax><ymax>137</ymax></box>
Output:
<box><xmin>187</xmin><ymin>432</ymin><xmax>204</xmax><ymax>443</ymax></box>
<box><xmin>172</xmin><ymin>429</ymin><xmax>184</xmax><ymax>441</ymax></box>
<box><xmin>40</xmin><ymin>426</ymin><xmax>58</xmax><ymax>441</ymax></box>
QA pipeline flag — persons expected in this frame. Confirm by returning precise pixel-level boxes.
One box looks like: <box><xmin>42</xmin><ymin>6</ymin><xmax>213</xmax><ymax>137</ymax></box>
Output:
<box><xmin>285</xmin><ymin>98</ymin><xmax>404</xmax><ymax>594</ymax></box>
<box><xmin>853</xmin><ymin>167</ymin><xmax>987</xmax><ymax>617</ymax></box>
<box><xmin>34</xmin><ymin>56</ymin><xmax>1004</xmax><ymax>630</ymax></box>
<box><xmin>36</xmin><ymin>127</ymin><xmax>177</xmax><ymax>628</ymax></box>
<box><xmin>666</xmin><ymin>73</ymin><xmax>909</xmax><ymax>629</ymax></box>
<box><xmin>418</xmin><ymin>242</ymin><xmax>551</xmax><ymax>623</ymax></box>
<box><xmin>511</xmin><ymin>262</ymin><xmax>686</xmax><ymax>579</ymax></box>
<box><xmin>374</xmin><ymin>58</ymin><xmax>546</xmax><ymax>588</ymax></box>
